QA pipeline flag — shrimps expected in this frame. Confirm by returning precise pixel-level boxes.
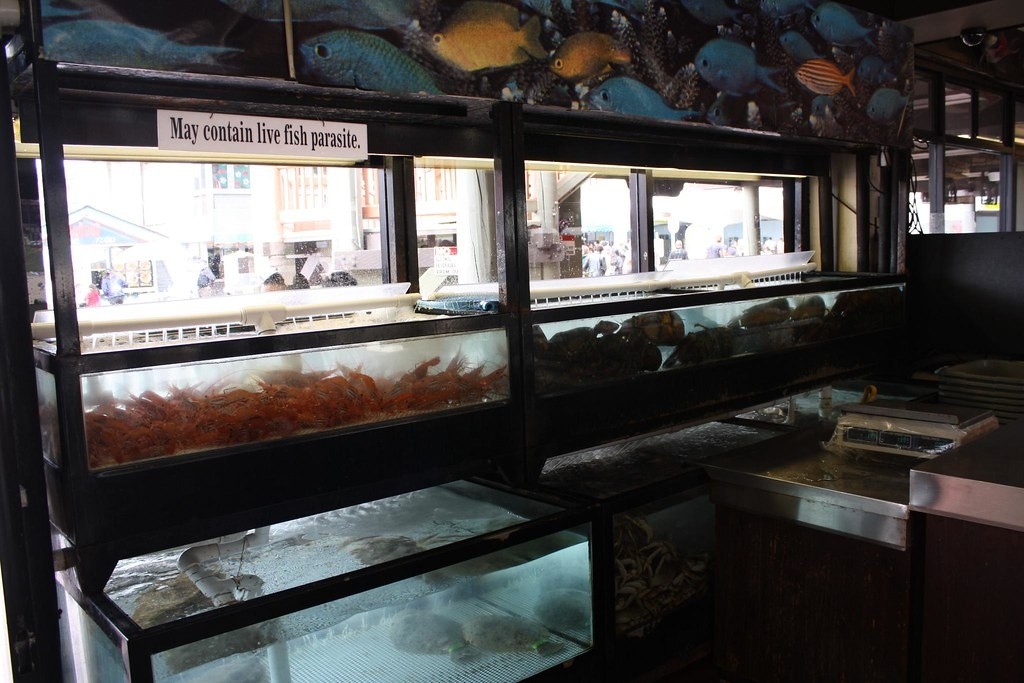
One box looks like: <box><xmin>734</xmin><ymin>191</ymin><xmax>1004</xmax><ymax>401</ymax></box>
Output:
<box><xmin>38</xmin><ymin>357</ymin><xmax>507</xmax><ymax>468</ymax></box>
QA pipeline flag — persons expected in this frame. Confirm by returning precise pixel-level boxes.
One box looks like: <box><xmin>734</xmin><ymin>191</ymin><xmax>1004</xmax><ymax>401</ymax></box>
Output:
<box><xmin>101</xmin><ymin>268</ymin><xmax>127</xmax><ymax>304</ymax></box>
<box><xmin>85</xmin><ymin>283</ymin><xmax>100</xmax><ymax>306</ymax></box>
<box><xmin>264</xmin><ymin>271</ymin><xmax>357</xmax><ymax>294</ymax></box>
<box><xmin>196</xmin><ymin>259</ymin><xmax>216</xmax><ymax>298</ymax></box>
<box><xmin>582</xmin><ymin>237</ymin><xmax>784</xmax><ymax>278</ymax></box>
